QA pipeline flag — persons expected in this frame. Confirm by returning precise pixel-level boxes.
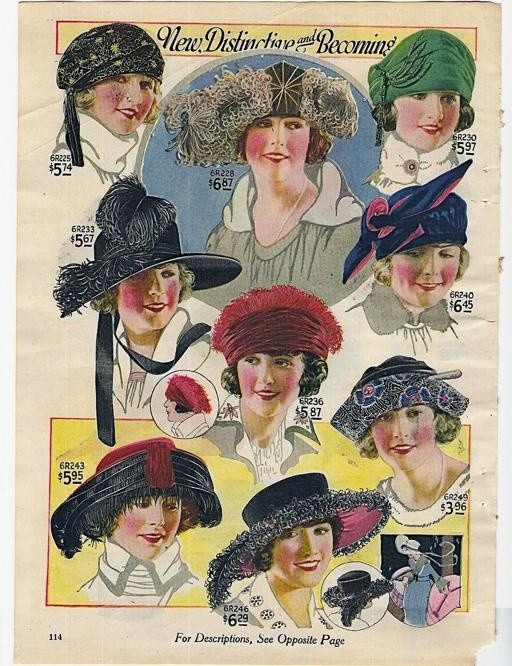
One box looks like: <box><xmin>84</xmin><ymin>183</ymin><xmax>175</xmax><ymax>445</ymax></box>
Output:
<box><xmin>160</xmin><ymin>374</ymin><xmax>213</xmax><ymax>440</ymax></box>
<box><xmin>328</xmin><ymin>354</ymin><xmax>468</xmax><ymax>527</ymax></box>
<box><xmin>196</xmin><ymin>283</ymin><xmax>342</xmax><ymax>486</ymax></box>
<box><xmin>87</xmin><ymin>263</ymin><xmax>210</xmax><ymax>413</ymax></box>
<box><xmin>202</xmin><ymin>471</ymin><xmax>389</xmax><ymax>634</ymax></box>
<box><xmin>49</xmin><ymin>21</ymin><xmax>163</xmax><ymax>185</ymax></box>
<box><xmin>47</xmin><ymin>438</ymin><xmax>222</xmax><ymax>610</ymax></box>
<box><xmin>164</xmin><ymin>59</ymin><xmax>375</xmax><ymax>314</ymax></box>
<box><xmin>368</xmin><ymin>26</ymin><xmax>473</xmax><ymax>194</ymax></box>
<box><xmin>341</xmin><ymin>160</ymin><xmax>471</xmax><ymax>352</ymax></box>
<box><xmin>395</xmin><ymin>536</ymin><xmax>446</xmax><ymax>626</ymax></box>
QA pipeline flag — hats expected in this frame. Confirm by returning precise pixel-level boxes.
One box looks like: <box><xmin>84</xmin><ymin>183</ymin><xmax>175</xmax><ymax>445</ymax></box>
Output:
<box><xmin>332</xmin><ymin>356</ymin><xmax>466</xmax><ymax>440</ymax></box>
<box><xmin>168</xmin><ymin>63</ymin><xmax>357</xmax><ymax>166</ymax></box>
<box><xmin>166</xmin><ymin>374</ymin><xmax>211</xmax><ymax>413</ymax></box>
<box><xmin>321</xmin><ymin>569</ymin><xmax>394</xmax><ymax>627</ymax></box>
<box><xmin>50</xmin><ymin>438</ymin><xmax>221</xmax><ymax>560</ymax></box>
<box><xmin>368</xmin><ymin>28</ymin><xmax>474</xmax><ymax>106</ymax></box>
<box><xmin>343</xmin><ymin>159</ymin><xmax>475</xmax><ymax>286</ymax></box>
<box><xmin>204</xmin><ymin>472</ymin><xmax>392</xmax><ymax>608</ymax></box>
<box><xmin>57</xmin><ymin>21</ymin><xmax>165</xmax><ymax>91</ymax></box>
<box><xmin>394</xmin><ymin>534</ymin><xmax>436</xmax><ymax>556</ymax></box>
<box><xmin>211</xmin><ymin>284</ymin><xmax>345</xmax><ymax>366</ymax></box>
<box><xmin>55</xmin><ymin>174</ymin><xmax>242</xmax><ymax>315</ymax></box>
